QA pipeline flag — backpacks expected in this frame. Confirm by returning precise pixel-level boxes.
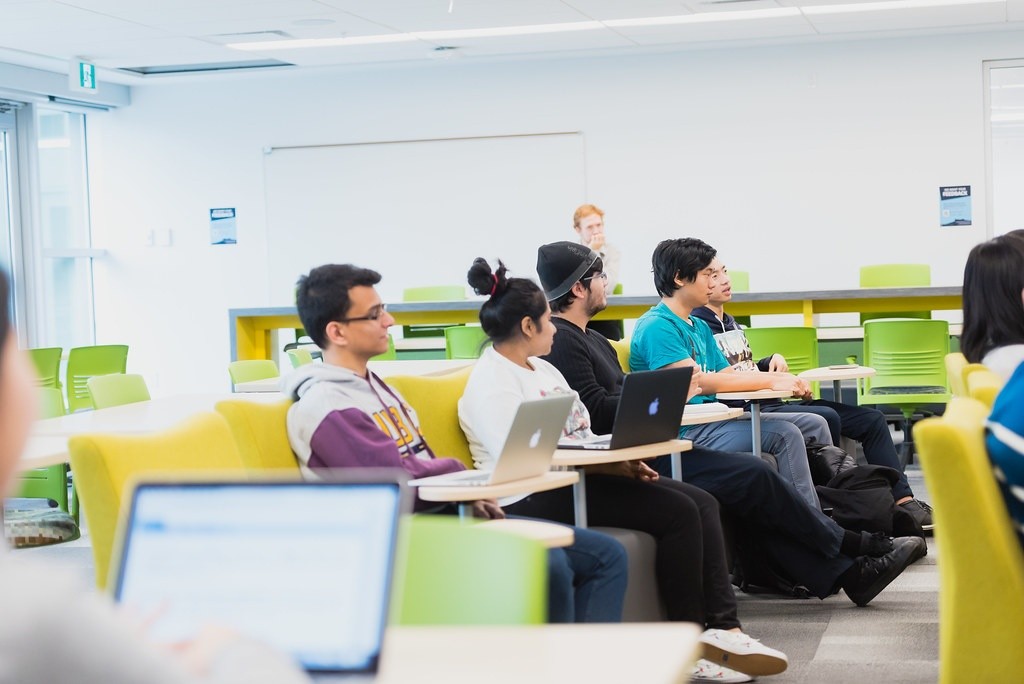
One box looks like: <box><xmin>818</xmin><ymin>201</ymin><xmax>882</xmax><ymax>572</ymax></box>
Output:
<box><xmin>804</xmin><ymin>435</ymin><xmax>858</xmax><ymax>485</ymax></box>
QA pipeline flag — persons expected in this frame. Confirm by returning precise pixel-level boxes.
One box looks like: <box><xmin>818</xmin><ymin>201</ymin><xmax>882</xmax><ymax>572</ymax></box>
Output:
<box><xmin>693</xmin><ymin>257</ymin><xmax>933</xmax><ymax>534</ymax></box>
<box><xmin>458</xmin><ymin>258</ymin><xmax>787</xmax><ymax>683</ymax></box>
<box><xmin>287</xmin><ymin>263</ymin><xmax>628</xmax><ymax>623</ymax></box>
<box><xmin>961</xmin><ymin>230</ymin><xmax>1023</xmax><ymax>549</ymax></box>
<box><xmin>0</xmin><ymin>273</ymin><xmax>310</xmax><ymax>684</ymax></box>
<box><xmin>573</xmin><ymin>205</ymin><xmax>621</xmax><ymax>341</ymax></box>
<box><xmin>536</xmin><ymin>242</ymin><xmax>927</xmax><ymax>606</ymax></box>
<box><xmin>628</xmin><ymin>237</ymin><xmax>833</xmax><ymax>512</ymax></box>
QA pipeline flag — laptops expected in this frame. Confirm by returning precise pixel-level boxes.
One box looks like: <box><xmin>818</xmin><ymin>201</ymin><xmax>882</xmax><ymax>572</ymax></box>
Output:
<box><xmin>408</xmin><ymin>395</ymin><xmax>578</xmax><ymax>486</ymax></box>
<box><xmin>104</xmin><ymin>468</ymin><xmax>416</xmax><ymax>684</ymax></box>
<box><xmin>557</xmin><ymin>366</ymin><xmax>694</xmax><ymax>451</ymax></box>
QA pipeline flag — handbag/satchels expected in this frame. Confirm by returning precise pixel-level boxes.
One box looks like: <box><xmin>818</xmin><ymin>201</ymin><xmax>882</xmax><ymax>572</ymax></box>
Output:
<box><xmin>814</xmin><ymin>464</ymin><xmax>895</xmax><ymax>540</ymax></box>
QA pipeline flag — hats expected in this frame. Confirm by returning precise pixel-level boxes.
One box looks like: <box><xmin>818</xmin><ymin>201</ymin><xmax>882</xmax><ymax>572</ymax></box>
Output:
<box><xmin>537</xmin><ymin>241</ymin><xmax>598</xmax><ymax>302</ymax></box>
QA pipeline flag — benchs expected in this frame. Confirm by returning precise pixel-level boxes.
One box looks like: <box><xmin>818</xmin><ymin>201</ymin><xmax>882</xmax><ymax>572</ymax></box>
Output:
<box><xmin>71</xmin><ymin>336</ymin><xmax>630</xmax><ymax>594</ymax></box>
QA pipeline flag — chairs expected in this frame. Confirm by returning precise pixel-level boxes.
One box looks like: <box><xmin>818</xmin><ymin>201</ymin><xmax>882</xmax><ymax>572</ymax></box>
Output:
<box><xmin>393</xmin><ymin>515</ymin><xmax>552</xmax><ymax>619</ymax></box>
<box><xmin>15</xmin><ymin>345</ymin><xmax>151</xmax><ymax>516</ymax></box>
<box><xmin>226</xmin><ymin>264</ymin><xmax>953</xmax><ymax>475</ymax></box>
<box><xmin>913</xmin><ymin>352</ymin><xmax>1024</xmax><ymax>684</ymax></box>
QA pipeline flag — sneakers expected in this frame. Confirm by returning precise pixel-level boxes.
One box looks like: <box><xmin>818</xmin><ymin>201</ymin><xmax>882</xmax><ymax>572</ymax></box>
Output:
<box><xmin>860</xmin><ymin>530</ymin><xmax>925</xmax><ymax>565</ymax></box>
<box><xmin>698</xmin><ymin>628</ymin><xmax>787</xmax><ymax>677</ymax></box>
<box><xmin>841</xmin><ymin>540</ymin><xmax>920</xmax><ymax>607</ymax></box>
<box><xmin>899</xmin><ymin>499</ymin><xmax>934</xmax><ymax>530</ymax></box>
<box><xmin>690</xmin><ymin>658</ymin><xmax>754</xmax><ymax>684</ymax></box>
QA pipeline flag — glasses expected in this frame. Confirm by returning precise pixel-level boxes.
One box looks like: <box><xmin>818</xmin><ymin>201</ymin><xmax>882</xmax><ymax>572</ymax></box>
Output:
<box><xmin>575</xmin><ymin>271</ymin><xmax>606</xmax><ymax>284</ymax></box>
<box><xmin>322</xmin><ymin>303</ymin><xmax>385</xmax><ymax>329</ymax></box>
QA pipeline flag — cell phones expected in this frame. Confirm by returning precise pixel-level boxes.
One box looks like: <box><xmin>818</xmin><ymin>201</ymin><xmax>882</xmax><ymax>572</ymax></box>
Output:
<box><xmin>830</xmin><ymin>364</ymin><xmax>858</xmax><ymax>369</ymax></box>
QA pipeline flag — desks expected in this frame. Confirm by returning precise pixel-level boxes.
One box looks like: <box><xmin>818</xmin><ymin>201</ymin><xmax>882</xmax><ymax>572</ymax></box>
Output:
<box><xmin>227</xmin><ymin>287</ymin><xmax>966</xmax><ymax>393</ymax></box>
<box><xmin>16</xmin><ymin>359</ymin><xmax>477</xmax><ymax>471</ymax></box>
<box><xmin>379</xmin><ymin>620</ymin><xmax>705</xmax><ymax>684</ymax></box>
